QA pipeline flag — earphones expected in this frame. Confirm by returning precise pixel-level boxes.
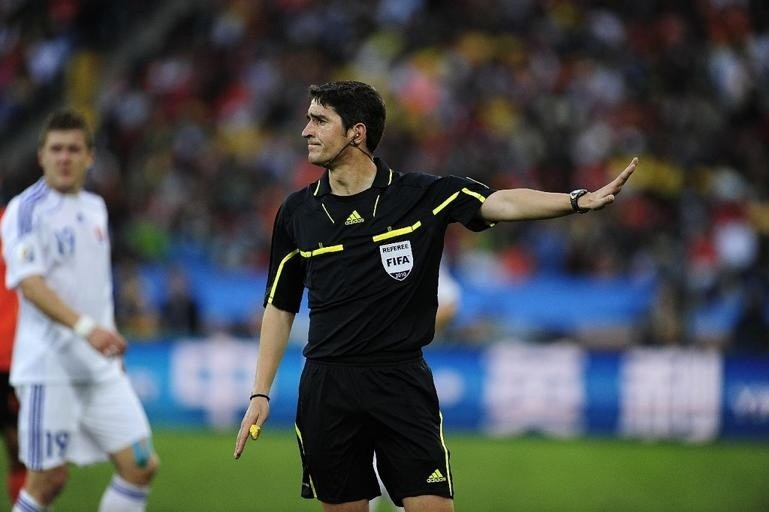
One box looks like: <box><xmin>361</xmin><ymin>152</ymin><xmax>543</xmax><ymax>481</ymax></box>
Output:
<box><xmin>351</xmin><ymin>132</ymin><xmax>360</xmax><ymax>141</ymax></box>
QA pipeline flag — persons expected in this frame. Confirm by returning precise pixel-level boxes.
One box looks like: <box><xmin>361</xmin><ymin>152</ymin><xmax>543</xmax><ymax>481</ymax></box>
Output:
<box><xmin>0</xmin><ymin>208</ymin><xmax>26</xmax><ymax>505</ymax></box>
<box><xmin>0</xmin><ymin>105</ymin><xmax>159</xmax><ymax>512</ymax></box>
<box><xmin>233</xmin><ymin>78</ymin><xmax>639</xmax><ymax>512</ymax></box>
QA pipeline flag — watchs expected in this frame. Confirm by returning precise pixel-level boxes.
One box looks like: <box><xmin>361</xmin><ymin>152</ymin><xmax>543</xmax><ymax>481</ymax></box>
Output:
<box><xmin>569</xmin><ymin>189</ymin><xmax>588</xmax><ymax>214</ymax></box>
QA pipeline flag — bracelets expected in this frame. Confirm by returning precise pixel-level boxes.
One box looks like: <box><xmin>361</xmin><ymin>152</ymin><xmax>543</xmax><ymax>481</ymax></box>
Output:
<box><xmin>70</xmin><ymin>314</ymin><xmax>100</xmax><ymax>342</ymax></box>
<box><xmin>250</xmin><ymin>394</ymin><xmax>271</xmax><ymax>400</ymax></box>
